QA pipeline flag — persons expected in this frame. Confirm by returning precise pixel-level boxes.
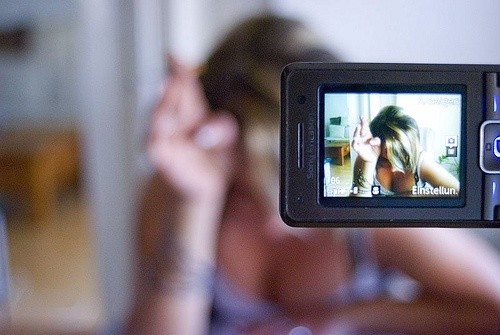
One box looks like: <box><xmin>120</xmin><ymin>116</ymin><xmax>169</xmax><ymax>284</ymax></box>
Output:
<box><xmin>349</xmin><ymin>105</ymin><xmax>460</xmax><ymax>197</ymax></box>
<box><xmin>124</xmin><ymin>15</ymin><xmax>500</xmax><ymax>335</ymax></box>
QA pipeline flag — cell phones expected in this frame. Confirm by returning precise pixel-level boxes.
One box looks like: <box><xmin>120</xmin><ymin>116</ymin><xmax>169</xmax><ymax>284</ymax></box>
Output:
<box><xmin>280</xmin><ymin>61</ymin><xmax>499</xmax><ymax>229</ymax></box>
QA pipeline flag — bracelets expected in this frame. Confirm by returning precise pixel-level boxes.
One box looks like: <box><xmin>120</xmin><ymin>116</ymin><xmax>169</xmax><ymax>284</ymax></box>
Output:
<box><xmin>352</xmin><ymin>169</ymin><xmax>375</xmax><ymax>193</ymax></box>
